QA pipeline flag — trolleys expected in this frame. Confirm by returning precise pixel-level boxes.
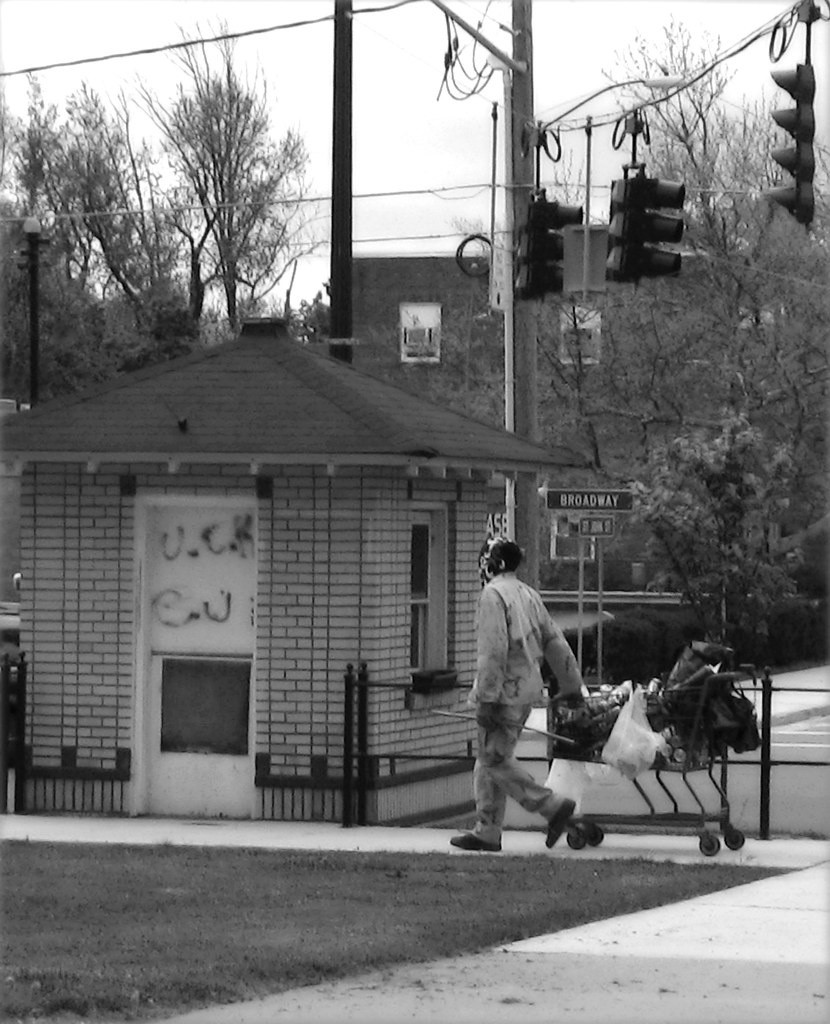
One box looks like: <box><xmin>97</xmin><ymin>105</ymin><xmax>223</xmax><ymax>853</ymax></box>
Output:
<box><xmin>543</xmin><ymin>664</ymin><xmax>758</xmax><ymax>857</ymax></box>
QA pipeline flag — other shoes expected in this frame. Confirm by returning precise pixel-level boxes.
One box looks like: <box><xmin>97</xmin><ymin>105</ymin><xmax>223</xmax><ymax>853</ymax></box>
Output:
<box><xmin>450</xmin><ymin>832</ymin><xmax>502</xmax><ymax>851</ymax></box>
<box><xmin>543</xmin><ymin>798</ymin><xmax>575</xmax><ymax>848</ymax></box>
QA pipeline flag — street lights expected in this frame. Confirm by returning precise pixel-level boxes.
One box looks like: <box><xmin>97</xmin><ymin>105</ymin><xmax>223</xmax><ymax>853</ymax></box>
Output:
<box><xmin>16</xmin><ymin>218</ymin><xmax>52</xmax><ymax>409</ymax></box>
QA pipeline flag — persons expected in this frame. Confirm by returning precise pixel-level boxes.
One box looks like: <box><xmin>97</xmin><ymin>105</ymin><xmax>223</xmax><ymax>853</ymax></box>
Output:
<box><xmin>450</xmin><ymin>536</ymin><xmax>576</xmax><ymax>848</ymax></box>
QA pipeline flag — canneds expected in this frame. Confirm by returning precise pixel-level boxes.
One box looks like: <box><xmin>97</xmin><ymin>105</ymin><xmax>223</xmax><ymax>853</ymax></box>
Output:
<box><xmin>555</xmin><ymin>676</ymin><xmax>686</xmax><ymax>765</ymax></box>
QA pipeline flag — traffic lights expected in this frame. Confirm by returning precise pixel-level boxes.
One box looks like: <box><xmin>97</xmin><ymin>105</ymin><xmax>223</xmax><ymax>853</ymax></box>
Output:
<box><xmin>604</xmin><ymin>167</ymin><xmax>683</xmax><ymax>283</ymax></box>
<box><xmin>766</xmin><ymin>66</ymin><xmax>817</xmax><ymax>226</ymax></box>
<box><xmin>521</xmin><ymin>201</ymin><xmax>582</xmax><ymax>292</ymax></box>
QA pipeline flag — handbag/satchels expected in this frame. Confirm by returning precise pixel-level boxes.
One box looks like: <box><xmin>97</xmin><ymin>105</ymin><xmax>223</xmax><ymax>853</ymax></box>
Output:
<box><xmin>703</xmin><ymin>670</ymin><xmax>761</xmax><ymax>756</ymax></box>
<box><xmin>601</xmin><ymin>684</ymin><xmax>666</xmax><ymax>780</ymax></box>
